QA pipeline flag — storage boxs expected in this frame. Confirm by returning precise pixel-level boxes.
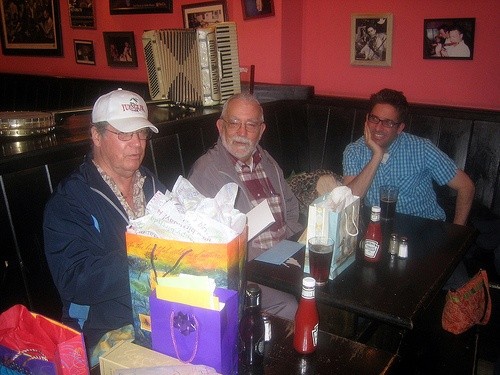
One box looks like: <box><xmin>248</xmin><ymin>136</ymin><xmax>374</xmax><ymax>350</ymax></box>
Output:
<box><xmin>98</xmin><ymin>339</ymin><xmax>223</xmax><ymax>375</ymax></box>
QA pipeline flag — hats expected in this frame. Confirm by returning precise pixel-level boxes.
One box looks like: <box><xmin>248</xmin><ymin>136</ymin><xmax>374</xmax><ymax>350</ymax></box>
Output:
<box><xmin>91</xmin><ymin>88</ymin><xmax>159</xmax><ymax>134</ymax></box>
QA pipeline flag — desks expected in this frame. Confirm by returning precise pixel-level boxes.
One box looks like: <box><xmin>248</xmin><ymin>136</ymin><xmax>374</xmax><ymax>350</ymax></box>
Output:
<box><xmin>90</xmin><ymin>311</ymin><xmax>396</xmax><ymax>375</ymax></box>
<box><xmin>243</xmin><ymin>206</ymin><xmax>472</xmax><ymax>322</ymax></box>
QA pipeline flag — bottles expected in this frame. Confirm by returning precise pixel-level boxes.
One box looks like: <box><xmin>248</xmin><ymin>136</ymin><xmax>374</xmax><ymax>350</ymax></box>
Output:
<box><xmin>397</xmin><ymin>236</ymin><xmax>408</xmax><ymax>259</ymax></box>
<box><xmin>238</xmin><ymin>284</ymin><xmax>265</xmax><ymax>368</ymax></box>
<box><xmin>262</xmin><ymin>314</ymin><xmax>272</xmax><ymax>342</ymax></box>
<box><xmin>363</xmin><ymin>206</ymin><xmax>383</xmax><ymax>263</ymax></box>
<box><xmin>388</xmin><ymin>234</ymin><xmax>398</xmax><ymax>255</ymax></box>
<box><xmin>294</xmin><ymin>276</ymin><xmax>319</xmax><ymax>355</ymax></box>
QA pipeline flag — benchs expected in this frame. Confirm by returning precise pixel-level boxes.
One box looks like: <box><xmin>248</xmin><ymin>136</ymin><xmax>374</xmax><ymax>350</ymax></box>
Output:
<box><xmin>0</xmin><ymin>100</ymin><xmax>500</xmax><ymax>322</ymax></box>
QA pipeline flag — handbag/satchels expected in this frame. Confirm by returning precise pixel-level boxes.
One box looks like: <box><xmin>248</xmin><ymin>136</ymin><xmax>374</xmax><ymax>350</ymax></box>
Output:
<box><xmin>9</xmin><ymin>311</ymin><xmax>91</xmax><ymax>375</ymax></box>
<box><xmin>442</xmin><ymin>269</ymin><xmax>492</xmax><ymax>334</ymax></box>
<box><xmin>303</xmin><ymin>192</ymin><xmax>361</xmax><ymax>279</ymax></box>
<box><xmin>0</xmin><ymin>346</ymin><xmax>57</xmax><ymax>375</ymax></box>
<box><xmin>149</xmin><ymin>287</ymin><xmax>239</xmax><ymax>375</ymax></box>
<box><xmin>125</xmin><ymin>223</ymin><xmax>247</xmax><ymax>349</ymax></box>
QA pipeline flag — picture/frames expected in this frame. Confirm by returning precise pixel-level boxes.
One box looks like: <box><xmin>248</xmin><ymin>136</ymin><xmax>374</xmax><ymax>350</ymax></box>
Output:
<box><xmin>423</xmin><ymin>18</ymin><xmax>476</xmax><ymax>59</ymax></box>
<box><xmin>0</xmin><ymin>0</ymin><xmax>64</xmax><ymax>56</ymax></box>
<box><xmin>103</xmin><ymin>31</ymin><xmax>138</xmax><ymax>67</ymax></box>
<box><xmin>350</xmin><ymin>13</ymin><xmax>392</xmax><ymax>67</ymax></box>
<box><xmin>109</xmin><ymin>0</ymin><xmax>174</xmax><ymax>15</ymax></box>
<box><xmin>73</xmin><ymin>39</ymin><xmax>96</xmax><ymax>65</ymax></box>
<box><xmin>181</xmin><ymin>0</ymin><xmax>228</xmax><ymax>30</ymax></box>
<box><xmin>69</xmin><ymin>0</ymin><xmax>97</xmax><ymax>29</ymax></box>
<box><xmin>241</xmin><ymin>0</ymin><xmax>276</xmax><ymax>21</ymax></box>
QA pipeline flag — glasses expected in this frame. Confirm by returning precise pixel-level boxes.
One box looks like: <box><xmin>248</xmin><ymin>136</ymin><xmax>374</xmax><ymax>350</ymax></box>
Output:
<box><xmin>99</xmin><ymin>125</ymin><xmax>153</xmax><ymax>141</ymax></box>
<box><xmin>222</xmin><ymin>117</ymin><xmax>264</xmax><ymax>133</ymax></box>
<box><xmin>367</xmin><ymin>114</ymin><xmax>403</xmax><ymax>128</ymax></box>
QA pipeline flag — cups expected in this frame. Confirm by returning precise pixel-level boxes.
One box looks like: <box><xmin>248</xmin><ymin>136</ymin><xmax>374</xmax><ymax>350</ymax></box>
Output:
<box><xmin>380</xmin><ymin>185</ymin><xmax>399</xmax><ymax>222</ymax></box>
<box><xmin>308</xmin><ymin>236</ymin><xmax>335</xmax><ymax>287</ymax></box>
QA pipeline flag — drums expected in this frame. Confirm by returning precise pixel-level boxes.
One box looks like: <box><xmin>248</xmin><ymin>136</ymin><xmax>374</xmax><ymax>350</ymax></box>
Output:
<box><xmin>3</xmin><ymin>134</ymin><xmax>56</xmax><ymax>154</ymax></box>
<box><xmin>0</xmin><ymin>111</ymin><xmax>56</xmax><ymax>137</ymax></box>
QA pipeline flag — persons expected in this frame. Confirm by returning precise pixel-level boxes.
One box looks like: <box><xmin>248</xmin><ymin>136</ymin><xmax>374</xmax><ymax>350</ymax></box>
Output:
<box><xmin>358</xmin><ymin>24</ymin><xmax>384</xmax><ymax>61</ymax></box>
<box><xmin>109</xmin><ymin>42</ymin><xmax>131</xmax><ymax>61</ymax></box>
<box><xmin>343</xmin><ymin>88</ymin><xmax>475</xmax><ymax>227</ymax></box>
<box><xmin>188</xmin><ymin>93</ymin><xmax>305</xmax><ymax>321</ymax></box>
<box><xmin>432</xmin><ymin>28</ymin><xmax>470</xmax><ymax>57</ymax></box>
<box><xmin>43</xmin><ymin>89</ymin><xmax>173</xmax><ymax>356</ymax></box>
<box><xmin>6</xmin><ymin>2</ymin><xmax>54</xmax><ymax>43</ymax></box>
